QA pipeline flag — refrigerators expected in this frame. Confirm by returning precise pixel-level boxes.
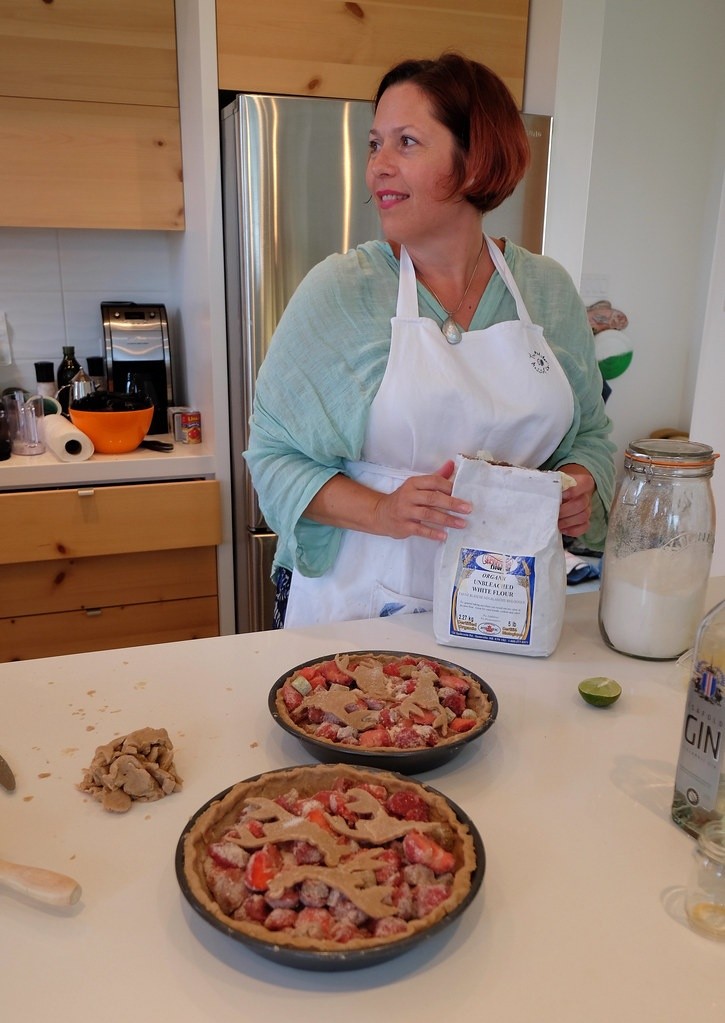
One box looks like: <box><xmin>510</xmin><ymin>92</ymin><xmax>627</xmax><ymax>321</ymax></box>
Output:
<box><xmin>221</xmin><ymin>93</ymin><xmax>552</xmax><ymax>636</ymax></box>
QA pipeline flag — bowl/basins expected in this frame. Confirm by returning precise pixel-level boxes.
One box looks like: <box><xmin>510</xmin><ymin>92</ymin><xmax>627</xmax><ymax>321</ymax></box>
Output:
<box><xmin>68</xmin><ymin>405</ymin><xmax>154</xmax><ymax>454</ymax></box>
<box><xmin>269</xmin><ymin>650</ymin><xmax>500</xmax><ymax>777</ymax></box>
<box><xmin>174</xmin><ymin>762</ymin><xmax>486</xmax><ymax>970</ymax></box>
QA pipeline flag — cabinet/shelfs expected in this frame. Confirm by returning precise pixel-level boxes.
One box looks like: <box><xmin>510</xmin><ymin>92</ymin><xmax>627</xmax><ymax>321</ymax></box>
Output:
<box><xmin>1</xmin><ymin>480</ymin><xmax>223</xmax><ymax>663</ymax></box>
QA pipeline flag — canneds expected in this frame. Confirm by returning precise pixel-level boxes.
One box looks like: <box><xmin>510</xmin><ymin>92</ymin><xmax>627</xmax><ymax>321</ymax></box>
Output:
<box><xmin>181</xmin><ymin>410</ymin><xmax>201</xmax><ymax>444</ymax></box>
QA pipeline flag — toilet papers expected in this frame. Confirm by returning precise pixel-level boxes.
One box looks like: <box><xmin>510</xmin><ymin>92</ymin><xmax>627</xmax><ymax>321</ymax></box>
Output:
<box><xmin>38</xmin><ymin>414</ymin><xmax>94</xmax><ymax>461</ymax></box>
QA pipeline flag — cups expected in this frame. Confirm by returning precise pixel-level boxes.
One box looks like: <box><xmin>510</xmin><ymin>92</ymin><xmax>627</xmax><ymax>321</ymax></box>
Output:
<box><xmin>2</xmin><ymin>393</ymin><xmax>45</xmax><ymax>456</ymax></box>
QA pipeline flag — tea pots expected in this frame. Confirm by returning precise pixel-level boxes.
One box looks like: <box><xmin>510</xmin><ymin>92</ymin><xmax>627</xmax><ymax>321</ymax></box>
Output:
<box><xmin>55</xmin><ymin>367</ymin><xmax>100</xmax><ymax>417</ymax></box>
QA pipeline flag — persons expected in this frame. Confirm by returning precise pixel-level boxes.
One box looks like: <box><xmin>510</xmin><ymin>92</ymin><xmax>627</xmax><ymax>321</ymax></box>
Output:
<box><xmin>243</xmin><ymin>52</ymin><xmax>616</xmax><ymax>630</ymax></box>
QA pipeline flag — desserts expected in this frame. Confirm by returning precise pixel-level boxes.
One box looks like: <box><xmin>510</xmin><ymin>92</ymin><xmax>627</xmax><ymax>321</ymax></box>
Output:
<box><xmin>275</xmin><ymin>654</ymin><xmax>493</xmax><ymax>751</ymax></box>
<box><xmin>183</xmin><ymin>765</ymin><xmax>475</xmax><ymax>952</ymax></box>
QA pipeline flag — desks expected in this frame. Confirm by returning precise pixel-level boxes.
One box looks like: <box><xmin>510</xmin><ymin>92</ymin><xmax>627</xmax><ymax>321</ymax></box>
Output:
<box><xmin>0</xmin><ymin>575</ymin><xmax>725</xmax><ymax>1022</ymax></box>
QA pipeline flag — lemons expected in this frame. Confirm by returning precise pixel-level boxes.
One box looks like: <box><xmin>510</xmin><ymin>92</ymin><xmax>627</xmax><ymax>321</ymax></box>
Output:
<box><xmin>695</xmin><ymin>902</ymin><xmax>725</xmax><ymax>933</ymax></box>
<box><xmin>578</xmin><ymin>676</ymin><xmax>622</xmax><ymax>709</ymax></box>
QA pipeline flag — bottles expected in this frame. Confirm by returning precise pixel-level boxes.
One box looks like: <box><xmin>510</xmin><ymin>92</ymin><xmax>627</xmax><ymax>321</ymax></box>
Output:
<box><xmin>57</xmin><ymin>346</ymin><xmax>81</xmax><ymax>420</ymax></box>
<box><xmin>86</xmin><ymin>356</ymin><xmax>106</xmax><ymax>392</ymax></box>
<box><xmin>684</xmin><ymin>819</ymin><xmax>725</xmax><ymax>936</ymax></box>
<box><xmin>34</xmin><ymin>362</ymin><xmax>55</xmax><ymax>399</ymax></box>
<box><xmin>597</xmin><ymin>438</ymin><xmax>720</xmax><ymax>661</ymax></box>
<box><xmin>671</xmin><ymin>599</ymin><xmax>725</xmax><ymax>839</ymax></box>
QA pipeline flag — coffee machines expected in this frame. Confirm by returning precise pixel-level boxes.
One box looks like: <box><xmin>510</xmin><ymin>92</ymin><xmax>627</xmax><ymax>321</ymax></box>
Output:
<box><xmin>100</xmin><ymin>301</ymin><xmax>176</xmax><ymax>436</ymax></box>
